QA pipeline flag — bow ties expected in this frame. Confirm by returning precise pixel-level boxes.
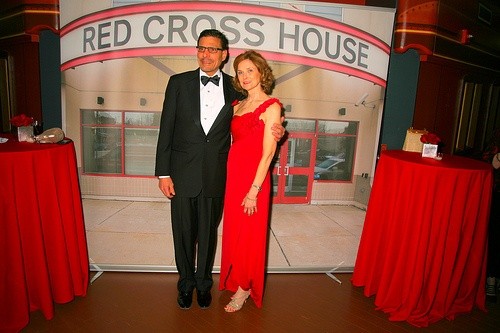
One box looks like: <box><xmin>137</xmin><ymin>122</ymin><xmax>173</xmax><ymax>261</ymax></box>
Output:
<box><xmin>201</xmin><ymin>75</ymin><xmax>220</xmax><ymax>86</ymax></box>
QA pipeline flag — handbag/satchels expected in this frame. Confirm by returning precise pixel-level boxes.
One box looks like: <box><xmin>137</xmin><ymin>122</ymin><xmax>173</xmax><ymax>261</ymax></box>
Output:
<box><xmin>34</xmin><ymin>128</ymin><xmax>64</xmax><ymax>144</ymax></box>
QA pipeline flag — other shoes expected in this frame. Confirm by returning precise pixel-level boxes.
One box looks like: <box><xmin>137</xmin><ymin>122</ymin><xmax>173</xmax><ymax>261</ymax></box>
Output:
<box><xmin>196</xmin><ymin>289</ymin><xmax>213</xmax><ymax>309</ymax></box>
<box><xmin>178</xmin><ymin>288</ymin><xmax>193</xmax><ymax>309</ymax></box>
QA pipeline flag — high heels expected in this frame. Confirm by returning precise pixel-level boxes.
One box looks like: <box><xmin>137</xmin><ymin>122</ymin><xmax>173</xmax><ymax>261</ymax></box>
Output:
<box><xmin>224</xmin><ymin>289</ymin><xmax>250</xmax><ymax>312</ymax></box>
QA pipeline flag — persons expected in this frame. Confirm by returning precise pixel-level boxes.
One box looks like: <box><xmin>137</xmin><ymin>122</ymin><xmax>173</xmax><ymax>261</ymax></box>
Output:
<box><xmin>220</xmin><ymin>50</ymin><xmax>283</xmax><ymax>311</ymax></box>
<box><xmin>482</xmin><ymin>111</ymin><xmax>500</xmax><ymax>302</ymax></box>
<box><xmin>154</xmin><ymin>29</ymin><xmax>285</xmax><ymax>308</ymax></box>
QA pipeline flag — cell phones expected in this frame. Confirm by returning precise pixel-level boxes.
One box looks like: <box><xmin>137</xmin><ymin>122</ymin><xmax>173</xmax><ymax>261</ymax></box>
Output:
<box><xmin>57</xmin><ymin>139</ymin><xmax>72</xmax><ymax>144</ymax></box>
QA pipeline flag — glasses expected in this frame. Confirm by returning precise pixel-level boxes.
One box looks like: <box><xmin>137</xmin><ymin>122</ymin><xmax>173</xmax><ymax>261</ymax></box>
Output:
<box><xmin>196</xmin><ymin>45</ymin><xmax>222</xmax><ymax>54</ymax></box>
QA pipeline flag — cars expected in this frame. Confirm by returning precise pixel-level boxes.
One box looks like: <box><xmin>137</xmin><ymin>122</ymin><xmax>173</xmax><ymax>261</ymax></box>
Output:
<box><xmin>313</xmin><ymin>152</ymin><xmax>346</xmax><ymax>180</ymax></box>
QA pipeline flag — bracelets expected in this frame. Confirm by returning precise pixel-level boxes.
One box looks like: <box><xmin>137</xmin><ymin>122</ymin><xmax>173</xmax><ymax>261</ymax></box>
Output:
<box><xmin>252</xmin><ymin>184</ymin><xmax>261</xmax><ymax>191</ymax></box>
<box><xmin>246</xmin><ymin>194</ymin><xmax>257</xmax><ymax>201</ymax></box>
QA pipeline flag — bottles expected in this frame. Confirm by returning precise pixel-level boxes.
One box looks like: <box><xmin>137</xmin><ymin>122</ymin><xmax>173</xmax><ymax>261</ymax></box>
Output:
<box><xmin>33</xmin><ymin>128</ymin><xmax>64</xmax><ymax>143</ymax></box>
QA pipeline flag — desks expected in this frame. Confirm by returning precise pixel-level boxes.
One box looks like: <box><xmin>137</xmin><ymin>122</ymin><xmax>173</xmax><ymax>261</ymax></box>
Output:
<box><xmin>0</xmin><ymin>134</ymin><xmax>89</xmax><ymax>333</ymax></box>
<box><xmin>349</xmin><ymin>149</ymin><xmax>493</xmax><ymax>327</ymax></box>
